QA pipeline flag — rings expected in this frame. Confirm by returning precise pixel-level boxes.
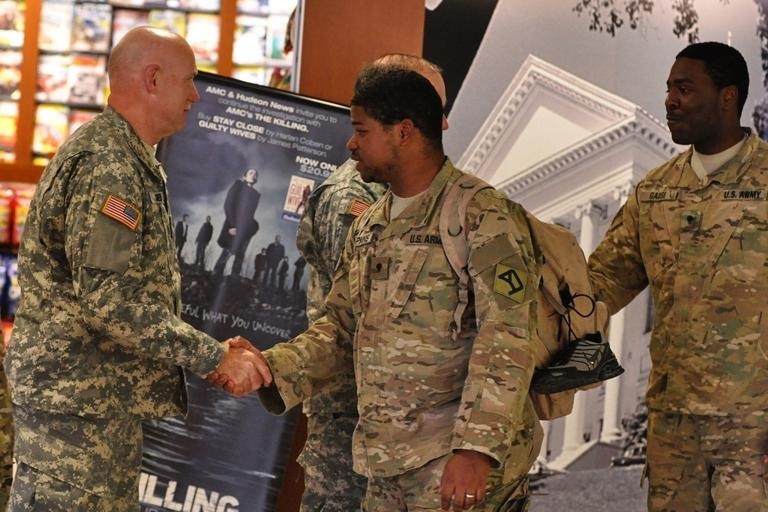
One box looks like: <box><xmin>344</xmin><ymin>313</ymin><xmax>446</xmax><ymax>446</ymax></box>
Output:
<box><xmin>460</xmin><ymin>488</ymin><xmax>481</xmax><ymax>501</ymax></box>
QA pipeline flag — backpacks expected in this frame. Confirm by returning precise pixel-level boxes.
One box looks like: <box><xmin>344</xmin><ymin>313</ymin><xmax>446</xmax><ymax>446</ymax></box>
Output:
<box><xmin>439</xmin><ymin>173</ymin><xmax>596</xmax><ymax>420</ymax></box>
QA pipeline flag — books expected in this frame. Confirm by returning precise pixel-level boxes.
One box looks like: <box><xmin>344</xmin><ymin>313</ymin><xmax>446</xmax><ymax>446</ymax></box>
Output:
<box><xmin>0</xmin><ymin>0</ymin><xmax>292</xmax><ymax>242</ymax></box>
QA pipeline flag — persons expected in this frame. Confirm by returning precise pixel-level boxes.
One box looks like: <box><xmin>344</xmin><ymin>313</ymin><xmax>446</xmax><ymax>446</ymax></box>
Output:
<box><xmin>0</xmin><ymin>324</ymin><xmax>14</xmax><ymax>510</ymax></box>
<box><xmin>174</xmin><ymin>169</ymin><xmax>312</xmax><ymax>297</ymax></box>
<box><xmin>207</xmin><ymin>71</ymin><xmax>542</xmax><ymax>512</ymax></box>
<box><xmin>298</xmin><ymin>55</ymin><xmax>450</xmax><ymax>510</ymax></box>
<box><xmin>587</xmin><ymin>43</ymin><xmax>767</xmax><ymax>511</ymax></box>
<box><xmin>7</xmin><ymin>25</ymin><xmax>273</xmax><ymax>511</ymax></box>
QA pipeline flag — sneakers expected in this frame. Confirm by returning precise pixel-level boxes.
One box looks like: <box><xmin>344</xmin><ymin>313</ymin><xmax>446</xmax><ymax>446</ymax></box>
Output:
<box><xmin>531</xmin><ymin>330</ymin><xmax>623</xmax><ymax>394</ymax></box>
